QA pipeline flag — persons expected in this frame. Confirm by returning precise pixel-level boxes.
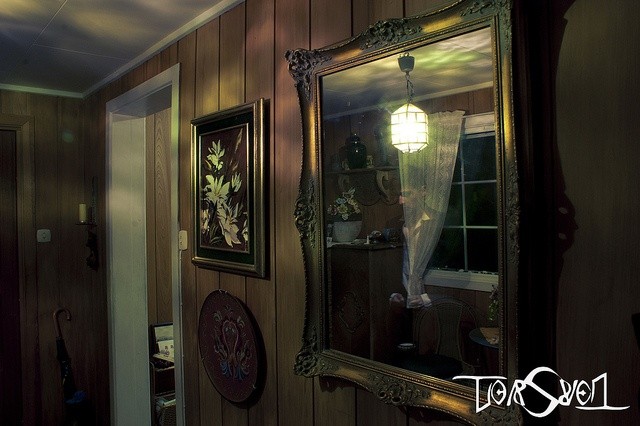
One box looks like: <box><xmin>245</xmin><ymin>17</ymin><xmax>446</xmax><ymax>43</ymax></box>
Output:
<box><xmin>383</xmin><ymin>167</ymin><xmax>446</xmax><ymax>351</ymax></box>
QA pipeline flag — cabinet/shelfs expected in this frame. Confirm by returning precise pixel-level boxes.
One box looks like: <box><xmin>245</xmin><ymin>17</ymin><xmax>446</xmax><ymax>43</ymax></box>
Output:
<box><xmin>329</xmin><ymin>241</ymin><xmax>412</xmax><ymax>367</ymax></box>
<box><xmin>149</xmin><ymin>359</ymin><xmax>175</xmax><ymax>426</ymax></box>
<box><xmin>327</xmin><ymin>156</ymin><xmax>399</xmax><ymax>207</ymax></box>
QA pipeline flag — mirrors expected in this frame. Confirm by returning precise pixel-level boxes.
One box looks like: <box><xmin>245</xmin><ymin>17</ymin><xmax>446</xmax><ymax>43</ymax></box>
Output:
<box><xmin>284</xmin><ymin>0</ymin><xmax>552</xmax><ymax>426</ymax></box>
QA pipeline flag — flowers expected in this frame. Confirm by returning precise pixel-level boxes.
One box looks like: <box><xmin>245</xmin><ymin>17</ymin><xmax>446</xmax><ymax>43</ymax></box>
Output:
<box><xmin>486</xmin><ymin>281</ymin><xmax>500</xmax><ymax>327</ymax></box>
<box><xmin>327</xmin><ymin>187</ymin><xmax>364</xmax><ymax>223</ymax></box>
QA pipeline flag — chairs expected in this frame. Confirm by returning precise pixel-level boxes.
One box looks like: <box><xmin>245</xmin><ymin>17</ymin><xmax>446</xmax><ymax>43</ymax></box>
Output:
<box><xmin>413</xmin><ymin>296</ymin><xmax>476</xmax><ymax>388</ymax></box>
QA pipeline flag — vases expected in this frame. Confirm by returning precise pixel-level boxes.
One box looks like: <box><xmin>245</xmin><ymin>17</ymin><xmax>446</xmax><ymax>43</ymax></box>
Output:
<box><xmin>329</xmin><ymin>220</ymin><xmax>363</xmax><ymax>241</ymax></box>
<box><xmin>468</xmin><ymin>328</ymin><xmax>501</xmax><ymax>360</ymax></box>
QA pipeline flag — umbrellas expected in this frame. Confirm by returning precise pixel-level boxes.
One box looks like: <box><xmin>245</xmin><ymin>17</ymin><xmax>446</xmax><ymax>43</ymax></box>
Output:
<box><xmin>53</xmin><ymin>308</ymin><xmax>81</xmax><ymax>425</ymax></box>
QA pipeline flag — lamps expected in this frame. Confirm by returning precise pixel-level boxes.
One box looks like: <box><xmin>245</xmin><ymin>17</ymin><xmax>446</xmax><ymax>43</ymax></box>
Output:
<box><xmin>391</xmin><ymin>52</ymin><xmax>429</xmax><ymax>153</ymax></box>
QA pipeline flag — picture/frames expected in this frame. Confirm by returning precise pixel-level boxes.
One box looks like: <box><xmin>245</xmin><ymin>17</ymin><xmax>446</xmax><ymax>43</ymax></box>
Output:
<box><xmin>189</xmin><ymin>96</ymin><xmax>266</xmax><ymax>279</ymax></box>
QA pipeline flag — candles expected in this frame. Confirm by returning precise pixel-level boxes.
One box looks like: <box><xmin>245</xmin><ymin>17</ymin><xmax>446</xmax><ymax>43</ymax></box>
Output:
<box><xmin>78</xmin><ymin>203</ymin><xmax>88</xmax><ymax>223</ymax></box>
